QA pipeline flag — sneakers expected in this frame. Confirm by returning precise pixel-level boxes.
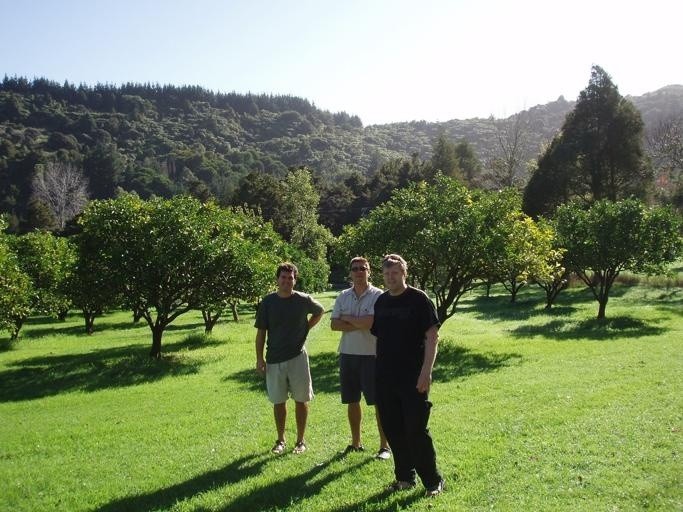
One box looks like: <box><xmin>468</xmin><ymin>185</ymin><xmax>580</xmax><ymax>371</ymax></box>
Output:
<box><xmin>388</xmin><ymin>475</ymin><xmax>447</xmax><ymax>497</ymax></box>
<box><xmin>343</xmin><ymin>442</ymin><xmax>392</xmax><ymax>461</ymax></box>
<box><xmin>273</xmin><ymin>440</ymin><xmax>307</xmax><ymax>455</ymax></box>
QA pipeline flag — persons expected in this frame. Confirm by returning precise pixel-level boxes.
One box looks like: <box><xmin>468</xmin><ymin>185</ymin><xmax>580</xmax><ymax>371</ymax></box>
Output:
<box><xmin>253</xmin><ymin>262</ymin><xmax>326</xmax><ymax>454</ymax></box>
<box><xmin>329</xmin><ymin>257</ymin><xmax>391</xmax><ymax>460</ymax></box>
<box><xmin>369</xmin><ymin>254</ymin><xmax>447</xmax><ymax>498</ymax></box>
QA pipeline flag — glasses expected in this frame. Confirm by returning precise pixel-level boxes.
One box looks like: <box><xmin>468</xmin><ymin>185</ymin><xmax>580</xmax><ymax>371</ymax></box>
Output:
<box><xmin>351</xmin><ymin>266</ymin><xmax>367</xmax><ymax>272</ymax></box>
